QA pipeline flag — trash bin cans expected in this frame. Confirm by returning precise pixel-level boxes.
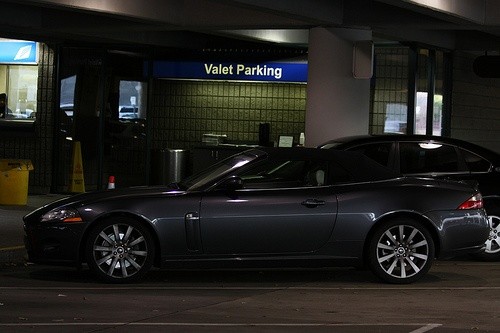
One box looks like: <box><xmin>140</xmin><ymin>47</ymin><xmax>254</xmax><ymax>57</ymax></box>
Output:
<box><xmin>156</xmin><ymin>148</ymin><xmax>190</xmax><ymax>187</ymax></box>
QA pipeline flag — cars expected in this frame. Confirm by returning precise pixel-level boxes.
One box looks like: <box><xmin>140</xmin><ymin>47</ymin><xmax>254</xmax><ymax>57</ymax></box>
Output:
<box><xmin>315</xmin><ymin>132</ymin><xmax>500</xmax><ymax>264</ymax></box>
<box><xmin>20</xmin><ymin>143</ymin><xmax>492</xmax><ymax>287</ymax></box>
